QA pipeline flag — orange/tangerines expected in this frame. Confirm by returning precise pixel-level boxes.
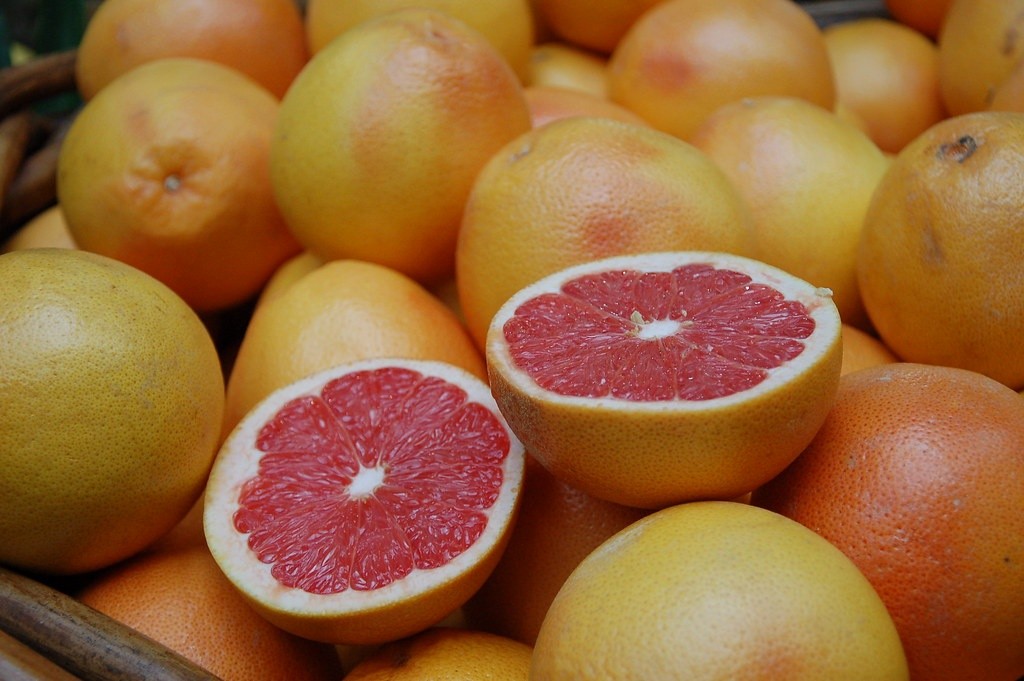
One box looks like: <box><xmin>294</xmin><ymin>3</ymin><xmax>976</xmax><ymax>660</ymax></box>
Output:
<box><xmin>0</xmin><ymin>0</ymin><xmax>1024</xmax><ymax>681</ymax></box>
